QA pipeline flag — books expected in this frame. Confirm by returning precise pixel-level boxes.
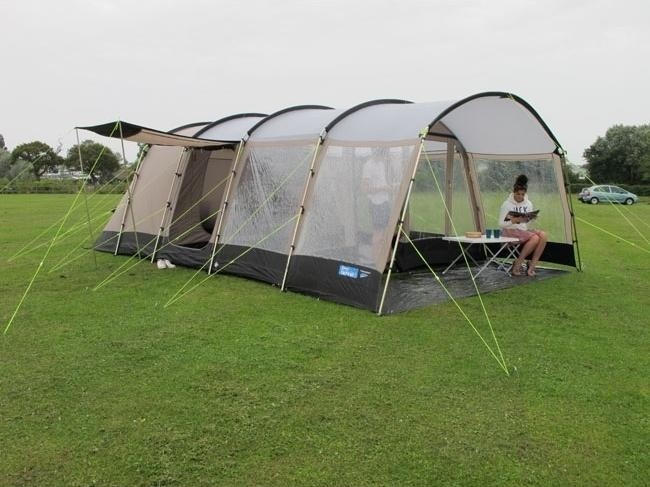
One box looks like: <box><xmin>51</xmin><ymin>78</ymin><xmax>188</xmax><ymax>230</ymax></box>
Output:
<box><xmin>504</xmin><ymin>208</ymin><xmax>540</xmax><ymax>225</ymax></box>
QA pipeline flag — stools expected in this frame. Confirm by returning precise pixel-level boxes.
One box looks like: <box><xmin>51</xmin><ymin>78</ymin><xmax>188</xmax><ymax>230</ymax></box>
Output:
<box><xmin>497</xmin><ymin>242</ymin><xmax>528</xmax><ymax>278</ymax></box>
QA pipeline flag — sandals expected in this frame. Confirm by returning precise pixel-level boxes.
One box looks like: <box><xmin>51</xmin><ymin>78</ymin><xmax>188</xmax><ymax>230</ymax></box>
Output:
<box><xmin>512</xmin><ymin>259</ymin><xmax>523</xmax><ymax>276</ymax></box>
<box><xmin>526</xmin><ymin>259</ymin><xmax>537</xmax><ymax>277</ymax></box>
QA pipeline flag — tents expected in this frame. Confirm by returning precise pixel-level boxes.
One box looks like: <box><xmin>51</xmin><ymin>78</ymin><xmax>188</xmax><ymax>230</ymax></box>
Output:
<box><xmin>70</xmin><ymin>89</ymin><xmax>578</xmax><ymax>320</ymax></box>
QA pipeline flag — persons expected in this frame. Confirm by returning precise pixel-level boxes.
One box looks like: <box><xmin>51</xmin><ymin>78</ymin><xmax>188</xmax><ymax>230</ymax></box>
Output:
<box><xmin>356</xmin><ymin>144</ymin><xmax>401</xmax><ymax>275</ymax></box>
<box><xmin>498</xmin><ymin>172</ymin><xmax>550</xmax><ymax>279</ymax></box>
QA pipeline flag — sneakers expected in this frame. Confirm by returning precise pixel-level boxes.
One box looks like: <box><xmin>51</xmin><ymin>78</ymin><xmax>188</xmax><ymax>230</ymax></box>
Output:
<box><xmin>156</xmin><ymin>258</ymin><xmax>177</xmax><ymax>270</ymax></box>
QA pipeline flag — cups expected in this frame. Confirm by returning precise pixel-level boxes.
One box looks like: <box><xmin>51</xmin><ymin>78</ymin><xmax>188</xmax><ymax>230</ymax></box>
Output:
<box><xmin>493</xmin><ymin>229</ymin><xmax>501</xmax><ymax>238</ymax></box>
<box><xmin>486</xmin><ymin>229</ymin><xmax>492</xmax><ymax>238</ymax></box>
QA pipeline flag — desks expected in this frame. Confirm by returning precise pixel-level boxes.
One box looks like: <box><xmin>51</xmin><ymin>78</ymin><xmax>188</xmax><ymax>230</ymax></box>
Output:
<box><xmin>442</xmin><ymin>234</ymin><xmax>520</xmax><ymax>280</ymax></box>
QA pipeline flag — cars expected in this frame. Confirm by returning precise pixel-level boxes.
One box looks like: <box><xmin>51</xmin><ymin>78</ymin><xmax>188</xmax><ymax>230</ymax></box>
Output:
<box><xmin>579</xmin><ymin>184</ymin><xmax>640</xmax><ymax>205</ymax></box>
